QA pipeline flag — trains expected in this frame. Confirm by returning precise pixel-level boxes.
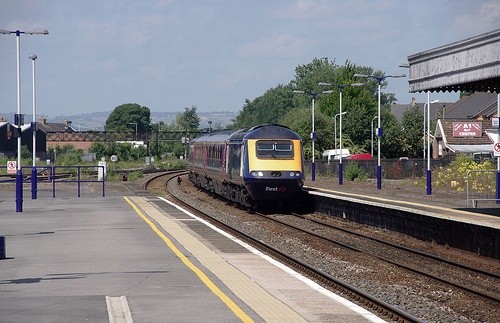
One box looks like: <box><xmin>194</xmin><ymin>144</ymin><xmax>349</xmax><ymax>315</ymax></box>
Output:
<box><xmin>188</xmin><ymin>123</ymin><xmax>304</xmax><ymax>211</ymax></box>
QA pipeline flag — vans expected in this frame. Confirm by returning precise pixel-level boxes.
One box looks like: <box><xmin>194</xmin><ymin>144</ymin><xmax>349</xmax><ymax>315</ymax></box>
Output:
<box><xmin>335</xmin><ymin>155</ymin><xmax>350</xmax><ymax>160</ymax></box>
<box><xmin>322</xmin><ymin>149</ymin><xmax>349</xmax><ymax>160</ymax></box>
<box><xmin>346</xmin><ymin>154</ymin><xmax>372</xmax><ymax>159</ymax></box>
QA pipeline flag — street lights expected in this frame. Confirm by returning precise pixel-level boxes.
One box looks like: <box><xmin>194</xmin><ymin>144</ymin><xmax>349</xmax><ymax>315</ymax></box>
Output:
<box><xmin>0</xmin><ymin>28</ymin><xmax>49</xmax><ymax>210</ymax></box>
<box><xmin>424</xmin><ymin>99</ymin><xmax>440</xmax><ymax>174</ymax></box>
<box><xmin>318</xmin><ymin>83</ymin><xmax>365</xmax><ymax>184</ymax></box>
<box><xmin>334</xmin><ymin>111</ymin><xmax>347</xmax><ymax>160</ymax></box>
<box><xmin>354</xmin><ymin>73</ymin><xmax>407</xmax><ymax>189</ymax></box>
<box><xmin>131</xmin><ymin>123</ymin><xmax>137</xmax><ymax>139</ymax></box>
<box><xmin>371</xmin><ymin>115</ymin><xmax>378</xmax><ymax>158</ymax></box>
<box><xmin>293</xmin><ymin>90</ymin><xmax>334</xmax><ymax>181</ymax></box>
<box><xmin>27</xmin><ymin>53</ymin><xmax>37</xmax><ymax>199</ymax></box>
